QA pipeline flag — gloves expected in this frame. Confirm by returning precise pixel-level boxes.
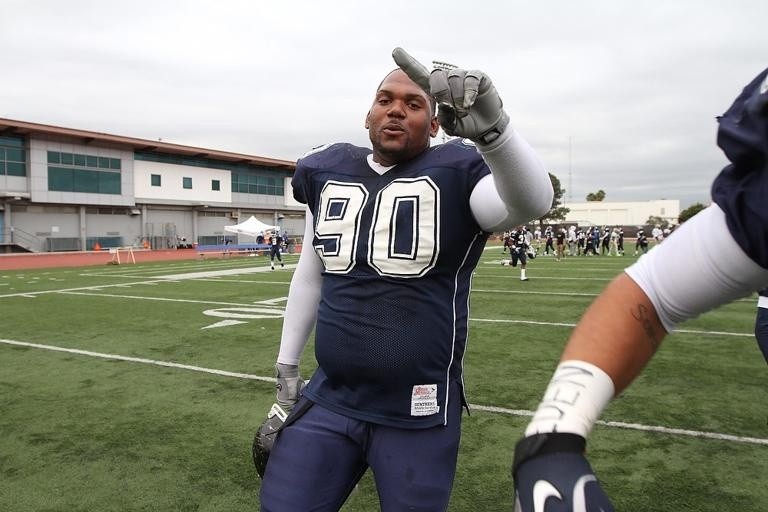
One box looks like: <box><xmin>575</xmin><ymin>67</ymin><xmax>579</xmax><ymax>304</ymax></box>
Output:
<box><xmin>276</xmin><ymin>363</ymin><xmax>306</xmax><ymax>412</ymax></box>
<box><xmin>393</xmin><ymin>48</ymin><xmax>509</xmax><ymax>146</ymax></box>
<box><xmin>514</xmin><ymin>432</ymin><xmax>615</xmax><ymax>511</ymax></box>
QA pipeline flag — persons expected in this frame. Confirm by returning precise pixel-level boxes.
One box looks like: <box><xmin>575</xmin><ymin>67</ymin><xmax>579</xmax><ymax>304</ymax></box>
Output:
<box><xmin>512</xmin><ymin>69</ymin><xmax>768</xmax><ymax>512</ymax></box>
<box><xmin>256</xmin><ymin>231</ymin><xmax>290</xmax><ymax>270</ymax></box>
<box><xmin>502</xmin><ymin>225</ymin><xmax>671</xmax><ymax>282</ymax></box>
<box><xmin>258</xmin><ymin>48</ymin><xmax>554</xmax><ymax>512</ymax></box>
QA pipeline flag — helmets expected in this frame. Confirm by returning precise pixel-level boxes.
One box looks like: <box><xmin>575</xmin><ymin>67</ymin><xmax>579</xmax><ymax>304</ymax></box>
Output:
<box><xmin>252</xmin><ymin>402</ymin><xmax>291</xmax><ymax>480</ymax></box>
